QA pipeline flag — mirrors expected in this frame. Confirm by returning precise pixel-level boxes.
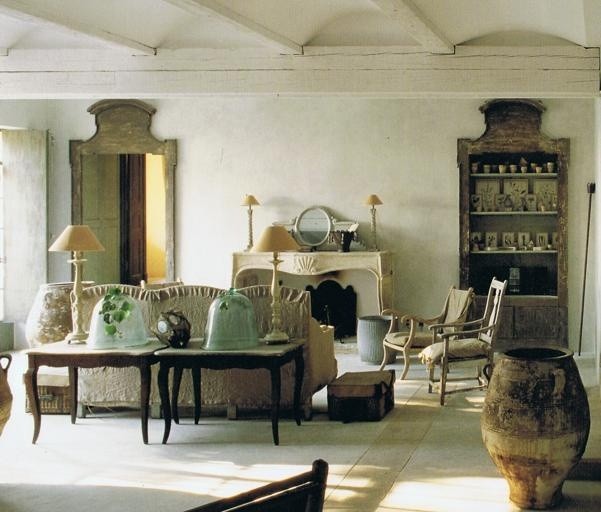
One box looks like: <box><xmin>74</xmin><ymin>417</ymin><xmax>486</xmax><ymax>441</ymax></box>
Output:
<box><xmin>293</xmin><ymin>207</ymin><xmax>332</xmax><ymax>247</ymax></box>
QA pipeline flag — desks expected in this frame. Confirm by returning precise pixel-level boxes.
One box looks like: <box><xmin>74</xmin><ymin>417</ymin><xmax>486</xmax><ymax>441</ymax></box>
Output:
<box><xmin>231</xmin><ymin>250</ymin><xmax>397</xmax><ymax>366</ymax></box>
<box><xmin>21</xmin><ymin>338</ymin><xmax>310</xmax><ymax>447</ymax></box>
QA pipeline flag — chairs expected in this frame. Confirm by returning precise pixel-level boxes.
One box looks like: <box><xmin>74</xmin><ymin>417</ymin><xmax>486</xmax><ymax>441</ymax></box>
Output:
<box><xmin>382</xmin><ymin>276</ymin><xmax>508</xmax><ymax>407</ymax></box>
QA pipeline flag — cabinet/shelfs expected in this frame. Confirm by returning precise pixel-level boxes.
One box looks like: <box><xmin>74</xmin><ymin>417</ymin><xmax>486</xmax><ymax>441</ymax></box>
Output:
<box><xmin>456</xmin><ymin>98</ymin><xmax>570</xmax><ymax>354</ymax></box>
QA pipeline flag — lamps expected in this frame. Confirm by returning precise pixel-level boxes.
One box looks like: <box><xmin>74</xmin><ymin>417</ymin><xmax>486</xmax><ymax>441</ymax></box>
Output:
<box><xmin>46</xmin><ymin>223</ymin><xmax>105</xmax><ymax>345</ymax></box>
<box><xmin>239</xmin><ymin>195</ymin><xmax>261</xmax><ymax>251</ymax></box>
<box><xmin>364</xmin><ymin>195</ymin><xmax>384</xmax><ymax>253</ymax></box>
<box><xmin>249</xmin><ymin>225</ymin><xmax>301</xmax><ymax>345</ymax></box>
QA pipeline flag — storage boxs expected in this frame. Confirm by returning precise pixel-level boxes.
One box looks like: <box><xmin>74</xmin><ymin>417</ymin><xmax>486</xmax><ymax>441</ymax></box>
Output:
<box><xmin>327</xmin><ymin>371</ymin><xmax>396</xmax><ymax>421</ymax></box>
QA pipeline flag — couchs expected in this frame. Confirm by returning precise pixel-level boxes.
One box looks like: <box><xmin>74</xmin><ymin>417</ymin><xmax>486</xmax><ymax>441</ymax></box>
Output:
<box><xmin>68</xmin><ymin>281</ymin><xmax>337</xmax><ymax>420</ymax></box>
